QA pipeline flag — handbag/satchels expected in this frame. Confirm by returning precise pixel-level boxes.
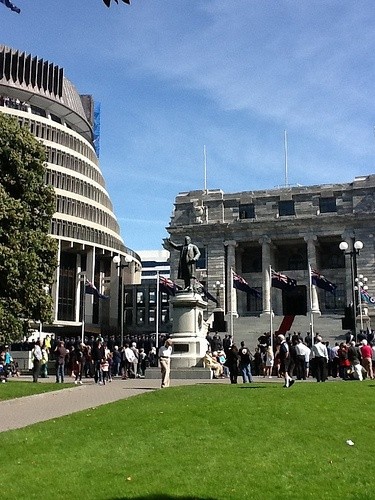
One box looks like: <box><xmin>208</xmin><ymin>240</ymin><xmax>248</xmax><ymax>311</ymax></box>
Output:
<box><xmin>341</xmin><ymin>358</ymin><xmax>350</xmax><ymax>366</ymax></box>
<box><xmin>248</xmin><ymin>352</ymin><xmax>255</xmax><ymax>361</ymax></box>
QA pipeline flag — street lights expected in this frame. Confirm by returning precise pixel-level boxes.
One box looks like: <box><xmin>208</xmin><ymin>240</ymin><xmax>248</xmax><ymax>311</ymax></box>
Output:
<box><xmin>212</xmin><ymin>281</ymin><xmax>224</xmax><ymax>308</ymax></box>
<box><xmin>354</xmin><ymin>274</ymin><xmax>369</xmax><ymax>331</ymax></box>
<box><xmin>339</xmin><ymin>237</ymin><xmax>363</xmax><ymax>343</ymax></box>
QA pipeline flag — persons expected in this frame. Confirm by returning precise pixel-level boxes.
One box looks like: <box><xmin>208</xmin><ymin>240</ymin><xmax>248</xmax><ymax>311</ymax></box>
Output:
<box><xmin>168</xmin><ymin>235</ymin><xmax>200</xmax><ymax>292</ymax></box>
<box><xmin>0</xmin><ymin>332</ymin><xmax>168</xmax><ymax>385</ymax></box>
<box><xmin>348</xmin><ymin>300</ymin><xmax>354</xmax><ymax>318</ymax></box>
<box><xmin>202</xmin><ymin>330</ymin><xmax>375</xmax><ymax>388</ymax></box>
<box><xmin>158</xmin><ymin>339</ymin><xmax>173</xmax><ymax>389</ymax></box>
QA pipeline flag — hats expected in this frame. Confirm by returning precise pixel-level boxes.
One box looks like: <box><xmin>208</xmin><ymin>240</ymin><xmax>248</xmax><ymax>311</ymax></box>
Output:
<box><xmin>361</xmin><ymin>339</ymin><xmax>367</xmax><ymax>343</ymax></box>
<box><xmin>131</xmin><ymin>342</ymin><xmax>136</xmax><ymax>347</ymax></box>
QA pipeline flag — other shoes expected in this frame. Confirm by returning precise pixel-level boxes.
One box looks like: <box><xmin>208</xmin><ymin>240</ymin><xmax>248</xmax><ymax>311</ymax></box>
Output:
<box><xmin>98</xmin><ymin>380</ymin><xmax>102</xmax><ymax>384</ymax></box>
<box><xmin>284</xmin><ymin>385</ymin><xmax>288</xmax><ymax>389</ymax></box>
<box><xmin>104</xmin><ymin>380</ymin><xmax>106</xmax><ymax>385</ymax></box>
<box><xmin>289</xmin><ymin>379</ymin><xmax>295</xmax><ymax>387</ymax></box>
<box><xmin>79</xmin><ymin>381</ymin><xmax>83</xmax><ymax>384</ymax></box>
<box><xmin>42</xmin><ymin>376</ymin><xmax>49</xmax><ymax>378</ymax></box>
<box><xmin>74</xmin><ymin>379</ymin><xmax>77</xmax><ymax>385</ymax></box>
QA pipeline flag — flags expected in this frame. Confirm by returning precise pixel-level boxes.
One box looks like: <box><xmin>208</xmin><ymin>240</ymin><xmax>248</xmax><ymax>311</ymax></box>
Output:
<box><xmin>270</xmin><ymin>270</ymin><xmax>296</xmax><ymax>289</ymax></box>
<box><xmin>158</xmin><ymin>275</ymin><xmax>178</xmax><ymax>296</ymax></box>
<box><xmin>83</xmin><ymin>280</ymin><xmax>111</xmax><ymax>302</ymax></box>
<box><xmin>309</xmin><ymin>268</ymin><xmax>337</xmax><ymax>294</ymax></box>
<box><xmin>198</xmin><ymin>281</ymin><xmax>219</xmax><ymax>306</ymax></box>
<box><xmin>231</xmin><ymin>271</ymin><xmax>261</xmax><ymax>300</ymax></box>
<box><xmin>359</xmin><ymin>286</ymin><xmax>375</xmax><ymax>307</ymax></box>
